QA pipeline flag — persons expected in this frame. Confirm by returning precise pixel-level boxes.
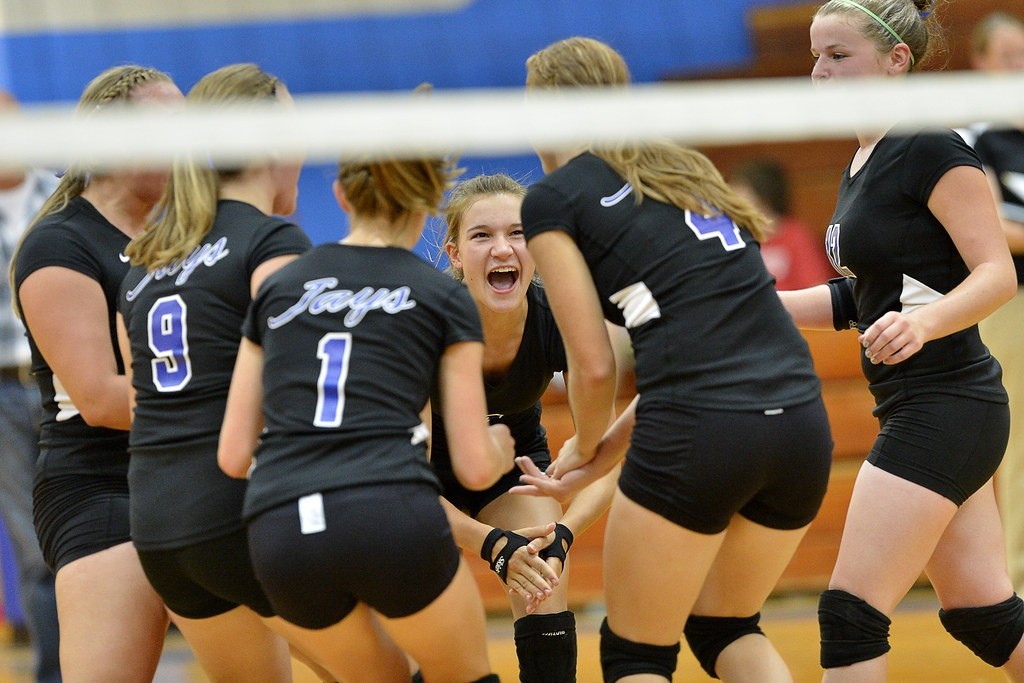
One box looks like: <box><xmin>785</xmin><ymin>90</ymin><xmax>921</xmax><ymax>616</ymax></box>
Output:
<box><xmin>215</xmin><ymin>159</ymin><xmax>520</xmax><ymax>682</ymax></box>
<box><xmin>417</xmin><ymin>170</ymin><xmax>626</xmax><ymax>683</ymax></box>
<box><xmin>952</xmin><ymin>12</ymin><xmax>1024</xmax><ymax>281</ymax></box>
<box><xmin>520</xmin><ymin>35</ymin><xmax>835</xmax><ymax>683</ymax></box>
<box><xmin>13</xmin><ymin>64</ymin><xmax>187</xmax><ymax>683</ymax></box>
<box><xmin>119</xmin><ymin>58</ymin><xmax>341</xmax><ymax>682</ymax></box>
<box><xmin>728</xmin><ymin>155</ymin><xmax>829</xmax><ymax>289</ymax></box>
<box><xmin>1</xmin><ymin>90</ymin><xmax>63</xmax><ymax>681</ymax></box>
<box><xmin>770</xmin><ymin>2</ymin><xmax>1024</xmax><ymax>683</ymax></box>
